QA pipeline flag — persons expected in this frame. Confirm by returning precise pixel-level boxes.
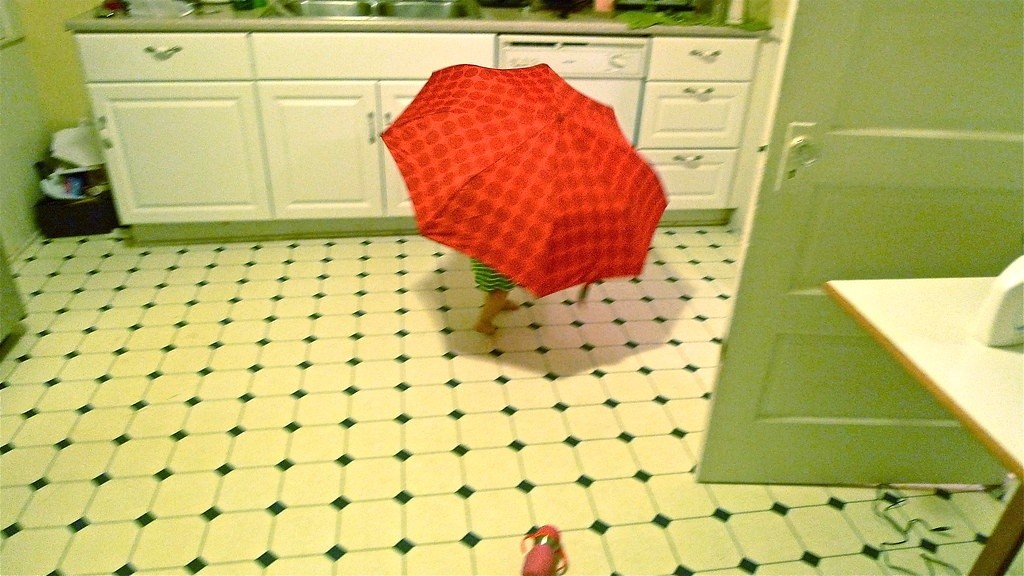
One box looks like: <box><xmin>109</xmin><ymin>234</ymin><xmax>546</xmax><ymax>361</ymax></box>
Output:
<box><xmin>470</xmin><ymin>256</ymin><xmax>519</xmax><ymax>334</ymax></box>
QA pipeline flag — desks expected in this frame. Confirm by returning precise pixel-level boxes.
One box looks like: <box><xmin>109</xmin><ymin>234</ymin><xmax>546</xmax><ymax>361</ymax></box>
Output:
<box><xmin>825</xmin><ymin>276</ymin><xmax>1024</xmax><ymax>576</ymax></box>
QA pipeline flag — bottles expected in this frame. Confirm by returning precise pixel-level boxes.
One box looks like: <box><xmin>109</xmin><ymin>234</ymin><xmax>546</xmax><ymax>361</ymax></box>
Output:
<box><xmin>521</xmin><ymin>525</ymin><xmax>568</xmax><ymax>576</ymax></box>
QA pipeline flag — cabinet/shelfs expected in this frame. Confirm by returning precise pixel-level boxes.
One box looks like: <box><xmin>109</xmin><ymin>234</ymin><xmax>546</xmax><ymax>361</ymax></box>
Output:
<box><xmin>75</xmin><ymin>35</ymin><xmax>780</xmax><ymax>243</ymax></box>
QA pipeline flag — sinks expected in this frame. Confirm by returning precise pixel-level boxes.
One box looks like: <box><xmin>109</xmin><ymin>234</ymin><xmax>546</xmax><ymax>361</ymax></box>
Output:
<box><xmin>375</xmin><ymin>0</ymin><xmax>485</xmax><ymax>20</ymax></box>
<box><xmin>255</xmin><ymin>0</ymin><xmax>373</xmax><ymax>16</ymax></box>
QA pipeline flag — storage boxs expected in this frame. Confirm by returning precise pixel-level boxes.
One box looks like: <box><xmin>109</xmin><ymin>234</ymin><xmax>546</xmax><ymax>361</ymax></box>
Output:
<box><xmin>31</xmin><ymin>192</ymin><xmax>117</xmax><ymax>238</ymax></box>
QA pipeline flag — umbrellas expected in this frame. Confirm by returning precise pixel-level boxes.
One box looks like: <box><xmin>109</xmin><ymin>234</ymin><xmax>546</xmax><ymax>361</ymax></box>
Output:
<box><xmin>380</xmin><ymin>63</ymin><xmax>670</xmax><ymax>299</ymax></box>
